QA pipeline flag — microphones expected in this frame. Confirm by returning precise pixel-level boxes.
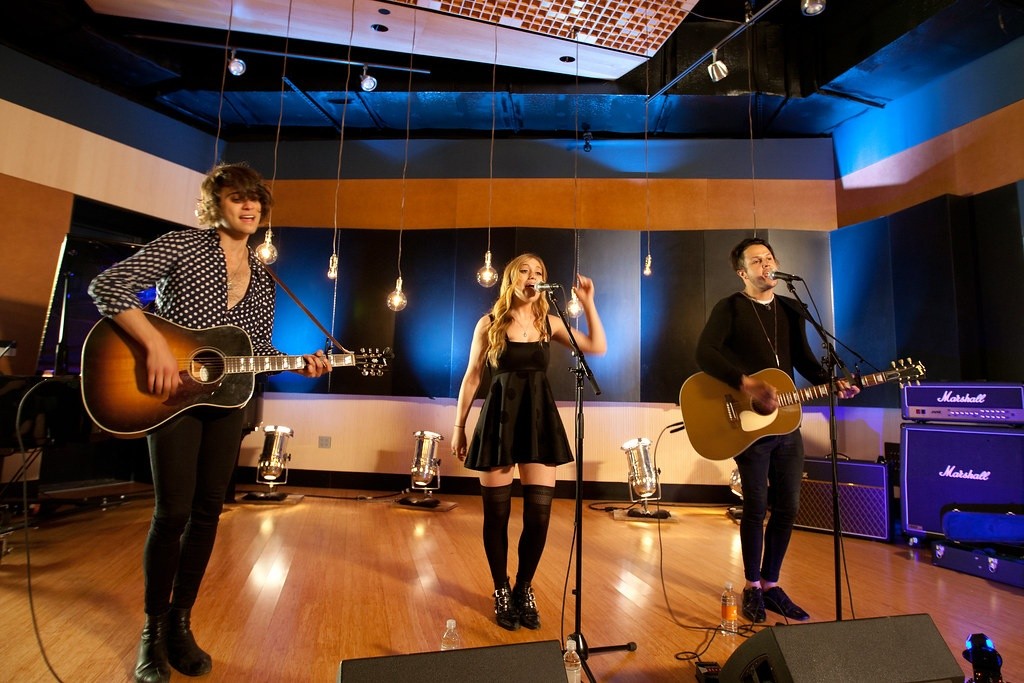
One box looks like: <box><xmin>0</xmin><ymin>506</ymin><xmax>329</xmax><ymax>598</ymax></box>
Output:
<box><xmin>534</xmin><ymin>280</ymin><xmax>562</xmax><ymax>292</ymax></box>
<box><xmin>769</xmin><ymin>270</ymin><xmax>803</xmax><ymax>281</ymax></box>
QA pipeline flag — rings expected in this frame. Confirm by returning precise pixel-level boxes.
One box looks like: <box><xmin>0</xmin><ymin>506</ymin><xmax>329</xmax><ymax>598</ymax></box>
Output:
<box><xmin>452</xmin><ymin>449</ymin><xmax>456</xmax><ymax>453</ymax></box>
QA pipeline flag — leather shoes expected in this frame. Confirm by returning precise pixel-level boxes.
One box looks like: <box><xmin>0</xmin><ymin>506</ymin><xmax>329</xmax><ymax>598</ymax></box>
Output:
<box><xmin>741</xmin><ymin>590</ymin><xmax>766</xmax><ymax>624</ymax></box>
<box><xmin>762</xmin><ymin>587</ymin><xmax>810</xmax><ymax>620</ymax></box>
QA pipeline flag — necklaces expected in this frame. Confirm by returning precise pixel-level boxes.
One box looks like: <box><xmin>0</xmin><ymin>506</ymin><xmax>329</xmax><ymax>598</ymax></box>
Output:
<box><xmin>226</xmin><ymin>247</ymin><xmax>246</xmax><ymax>289</ymax></box>
<box><xmin>510</xmin><ymin>307</ymin><xmax>533</xmax><ymax>338</ymax></box>
<box><xmin>745</xmin><ymin>292</ymin><xmax>780</xmax><ymax>368</ymax></box>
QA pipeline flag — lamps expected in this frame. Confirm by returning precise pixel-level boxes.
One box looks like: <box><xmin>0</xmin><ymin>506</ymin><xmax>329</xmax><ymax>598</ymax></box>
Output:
<box><xmin>707</xmin><ymin>49</ymin><xmax>728</xmax><ymax>83</ymax></box>
<box><xmin>360</xmin><ymin>66</ymin><xmax>377</xmax><ymax>91</ymax></box>
<box><xmin>228</xmin><ymin>50</ymin><xmax>246</xmax><ymax>76</ymax></box>
<box><xmin>234</xmin><ymin>425</ymin><xmax>304</xmax><ymax>504</ymax></box>
<box><xmin>388</xmin><ymin>431</ymin><xmax>458</xmax><ymax>512</ymax></box>
<box><xmin>613</xmin><ymin>438</ymin><xmax>680</xmax><ymax>523</ymax></box>
<box><xmin>800</xmin><ymin>0</ymin><xmax>826</xmax><ymax>16</ymax></box>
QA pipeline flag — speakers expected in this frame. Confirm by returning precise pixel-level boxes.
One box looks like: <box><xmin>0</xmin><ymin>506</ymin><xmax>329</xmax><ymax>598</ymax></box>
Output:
<box><xmin>338</xmin><ymin>638</ymin><xmax>568</xmax><ymax>683</ymax></box>
<box><xmin>719</xmin><ymin>614</ymin><xmax>965</xmax><ymax>683</ymax></box>
<box><xmin>791</xmin><ymin>458</ymin><xmax>895</xmax><ymax>541</ymax></box>
<box><xmin>899</xmin><ymin>423</ymin><xmax>1024</xmax><ymax>556</ymax></box>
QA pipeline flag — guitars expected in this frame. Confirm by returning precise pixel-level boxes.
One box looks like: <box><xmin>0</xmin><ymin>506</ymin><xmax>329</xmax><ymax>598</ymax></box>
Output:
<box><xmin>80</xmin><ymin>306</ymin><xmax>396</xmax><ymax>439</ymax></box>
<box><xmin>681</xmin><ymin>357</ymin><xmax>928</xmax><ymax>461</ymax></box>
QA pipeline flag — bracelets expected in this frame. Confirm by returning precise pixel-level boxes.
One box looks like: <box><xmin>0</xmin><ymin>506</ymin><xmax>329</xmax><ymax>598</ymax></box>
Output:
<box><xmin>454</xmin><ymin>424</ymin><xmax>465</xmax><ymax>428</ymax></box>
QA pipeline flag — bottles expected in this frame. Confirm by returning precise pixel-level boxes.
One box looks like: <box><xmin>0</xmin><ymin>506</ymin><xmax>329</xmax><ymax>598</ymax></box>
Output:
<box><xmin>563</xmin><ymin>640</ymin><xmax>581</xmax><ymax>683</ymax></box>
<box><xmin>721</xmin><ymin>581</ymin><xmax>737</xmax><ymax>636</ymax></box>
<box><xmin>441</xmin><ymin>618</ymin><xmax>460</xmax><ymax>651</ymax></box>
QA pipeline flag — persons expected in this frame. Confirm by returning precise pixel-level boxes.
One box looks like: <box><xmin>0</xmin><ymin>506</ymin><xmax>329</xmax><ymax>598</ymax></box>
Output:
<box><xmin>696</xmin><ymin>238</ymin><xmax>860</xmax><ymax>623</ymax></box>
<box><xmin>450</xmin><ymin>253</ymin><xmax>606</xmax><ymax>631</ymax></box>
<box><xmin>86</xmin><ymin>163</ymin><xmax>334</xmax><ymax>683</ymax></box>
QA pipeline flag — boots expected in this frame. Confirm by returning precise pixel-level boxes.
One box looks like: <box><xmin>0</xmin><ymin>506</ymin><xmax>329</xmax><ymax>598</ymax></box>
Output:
<box><xmin>135</xmin><ymin>613</ymin><xmax>170</xmax><ymax>683</ymax></box>
<box><xmin>166</xmin><ymin>606</ymin><xmax>211</xmax><ymax>676</ymax></box>
<box><xmin>513</xmin><ymin>574</ymin><xmax>540</xmax><ymax>630</ymax></box>
<box><xmin>492</xmin><ymin>578</ymin><xmax>516</xmax><ymax>630</ymax></box>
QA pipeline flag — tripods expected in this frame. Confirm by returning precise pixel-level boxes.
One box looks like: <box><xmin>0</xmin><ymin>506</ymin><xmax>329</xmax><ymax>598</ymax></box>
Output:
<box><xmin>549</xmin><ymin>291</ymin><xmax>636</xmax><ymax>683</ymax></box>
